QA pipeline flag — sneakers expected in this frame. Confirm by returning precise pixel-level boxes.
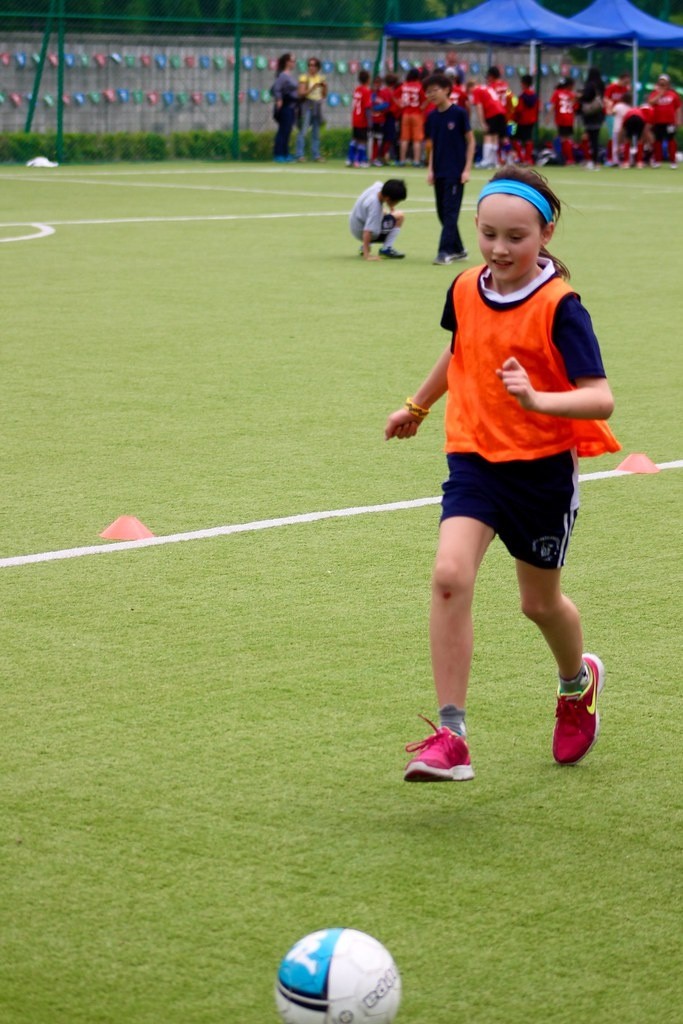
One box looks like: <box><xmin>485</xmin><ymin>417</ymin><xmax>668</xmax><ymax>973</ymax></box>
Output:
<box><xmin>552</xmin><ymin>653</ymin><xmax>607</xmax><ymax>767</ymax></box>
<box><xmin>378</xmin><ymin>247</ymin><xmax>406</xmax><ymax>260</ymax></box>
<box><xmin>402</xmin><ymin>713</ymin><xmax>476</xmax><ymax>783</ymax></box>
<box><xmin>433</xmin><ymin>251</ymin><xmax>470</xmax><ymax>266</ymax></box>
<box><xmin>359</xmin><ymin>246</ymin><xmax>372</xmax><ymax>256</ymax></box>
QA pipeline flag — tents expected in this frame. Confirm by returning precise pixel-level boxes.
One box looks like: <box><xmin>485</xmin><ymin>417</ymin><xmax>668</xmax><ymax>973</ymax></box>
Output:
<box><xmin>382</xmin><ymin>0</ymin><xmax>683</xmax><ymax>93</ymax></box>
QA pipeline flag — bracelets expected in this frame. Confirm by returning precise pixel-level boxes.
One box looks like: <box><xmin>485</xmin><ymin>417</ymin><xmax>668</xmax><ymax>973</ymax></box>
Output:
<box><xmin>404</xmin><ymin>397</ymin><xmax>430</xmax><ymax>418</ymax></box>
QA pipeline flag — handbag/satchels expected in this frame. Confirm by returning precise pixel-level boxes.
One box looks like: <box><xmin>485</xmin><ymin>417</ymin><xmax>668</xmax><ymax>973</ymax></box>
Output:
<box><xmin>580</xmin><ymin>80</ymin><xmax>603</xmax><ymax>117</ymax></box>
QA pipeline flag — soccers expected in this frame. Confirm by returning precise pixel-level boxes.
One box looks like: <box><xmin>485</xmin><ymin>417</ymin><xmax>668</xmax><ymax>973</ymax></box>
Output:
<box><xmin>275</xmin><ymin>927</ymin><xmax>401</xmax><ymax>1024</ymax></box>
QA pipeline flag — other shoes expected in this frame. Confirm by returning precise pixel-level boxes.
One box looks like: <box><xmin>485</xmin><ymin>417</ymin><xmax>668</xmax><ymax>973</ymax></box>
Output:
<box><xmin>604</xmin><ymin>162</ymin><xmax>612</xmax><ymax>169</ymax></box>
<box><xmin>360</xmin><ymin>162</ymin><xmax>371</xmax><ymax>168</ymax></box>
<box><xmin>585</xmin><ymin>161</ymin><xmax>594</xmax><ymax>170</ymax></box>
<box><xmin>390</xmin><ymin>160</ymin><xmax>397</xmax><ymax>167</ymax></box>
<box><xmin>650</xmin><ymin>162</ymin><xmax>663</xmax><ymax>170</ymax></box>
<box><xmin>518</xmin><ymin>161</ymin><xmax>534</xmax><ymax>168</ymax></box>
<box><xmin>315</xmin><ymin>157</ymin><xmax>326</xmax><ymax>163</ymax></box>
<box><xmin>345</xmin><ymin>159</ymin><xmax>352</xmax><ymax>167</ymax></box>
<box><xmin>412</xmin><ymin>161</ymin><xmax>422</xmax><ymax>167</ymax></box>
<box><xmin>669</xmin><ymin>163</ymin><xmax>678</xmax><ymax>170</ymax></box>
<box><xmin>298</xmin><ymin>156</ymin><xmax>307</xmax><ymax>163</ymax></box>
<box><xmin>398</xmin><ymin>161</ymin><xmax>406</xmax><ymax>167</ymax></box>
<box><xmin>274</xmin><ymin>155</ymin><xmax>295</xmax><ymax>164</ymax></box>
<box><xmin>373</xmin><ymin>159</ymin><xmax>384</xmax><ymax>167</ymax></box>
<box><xmin>636</xmin><ymin>162</ymin><xmax>647</xmax><ymax>169</ymax></box>
<box><xmin>565</xmin><ymin>160</ymin><xmax>577</xmax><ymax>167</ymax></box>
<box><xmin>621</xmin><ymin>162</ymin><xmax>632</xmax><ymax>170</ymax></box>
<box><xmin>474</xmin><ymin>163</ymin><xmax>499</xmax><ymax>171</ymax></box>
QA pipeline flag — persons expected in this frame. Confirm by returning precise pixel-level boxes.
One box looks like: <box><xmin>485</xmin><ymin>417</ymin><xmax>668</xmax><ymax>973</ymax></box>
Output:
<box><xmin>382</xmin><ymin>167</ymin><xmax>621</xmax><ymax>784</ymax></box>
<box><xmin>348</xmin><ymin>178</ymin><xmax>408</xmax><ymax>260</ymax></box>
<box><xmin>296</xmin><ymin>57</ymin><xmax>328</xmax><ymax>162</ymax></box>
<box><xmin>269</xmin><ymin>53</ymin><xmax>300</xmax><ymax>162</ymax></box>
<box><xmin>421</xmin><ymin>73</ymin><xmax>475</xmax><ymax>265</ymax></box>
<box><xmin>345</xmin><ymin>65</ymin><xmax>683</xmax><ymax>170</ymax></box>
